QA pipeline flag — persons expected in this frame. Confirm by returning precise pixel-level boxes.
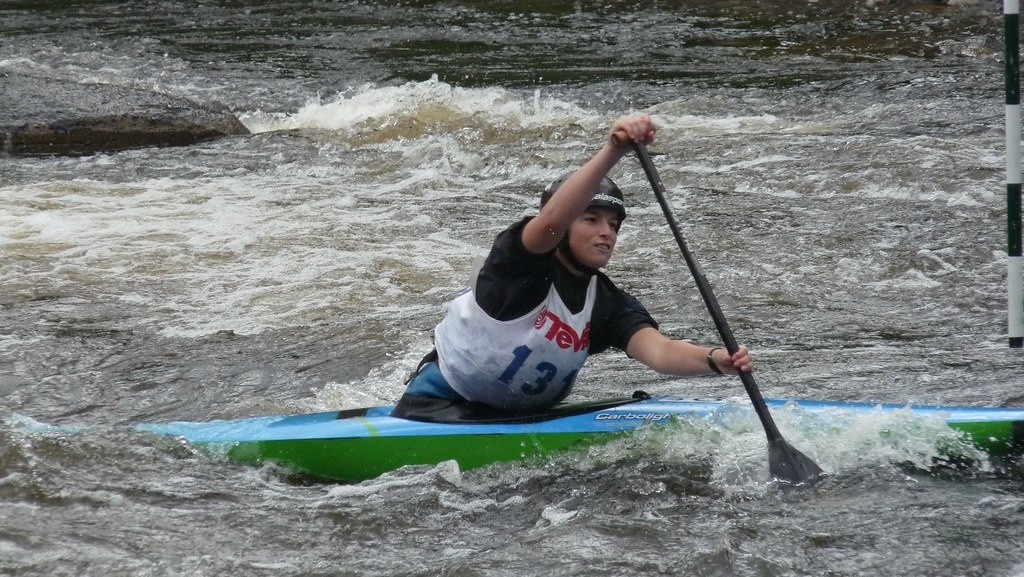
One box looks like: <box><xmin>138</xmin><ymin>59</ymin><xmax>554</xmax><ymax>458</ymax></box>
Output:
<box><xmin>390</xmin><ymin>111</ymin><xmax>755</xmax><ymax>423</ymax></box>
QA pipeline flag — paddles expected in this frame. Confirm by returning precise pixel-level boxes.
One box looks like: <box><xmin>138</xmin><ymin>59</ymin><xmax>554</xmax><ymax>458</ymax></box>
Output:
<box><xmin>613</xmin><ymin>129</ymin><xmax>823</xmax><ymax>482</ymax></box>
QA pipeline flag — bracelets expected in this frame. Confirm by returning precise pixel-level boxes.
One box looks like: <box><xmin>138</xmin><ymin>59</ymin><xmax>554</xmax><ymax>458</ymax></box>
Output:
<box><xmin>706</xmin><ymin>347</ymin><xmax>723</xmax><ymax>375</ymax></box>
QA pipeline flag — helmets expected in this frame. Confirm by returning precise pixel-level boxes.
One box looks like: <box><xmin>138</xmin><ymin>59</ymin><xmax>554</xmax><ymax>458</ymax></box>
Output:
<box><xmin>541</xmin><ymin>167</ymin><xmax>626</xmax><ymax>233</ymax></box>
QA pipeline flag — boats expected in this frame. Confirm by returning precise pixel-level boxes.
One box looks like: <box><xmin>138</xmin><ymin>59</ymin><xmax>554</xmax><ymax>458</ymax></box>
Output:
<box><xmin>132</xmin><ymin>387</ymin><xmax>1024</xmax><ymax>485</ymax></box>
